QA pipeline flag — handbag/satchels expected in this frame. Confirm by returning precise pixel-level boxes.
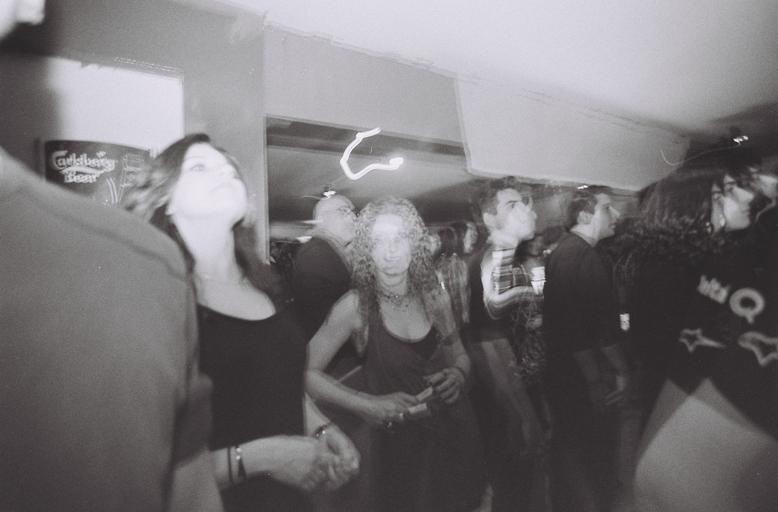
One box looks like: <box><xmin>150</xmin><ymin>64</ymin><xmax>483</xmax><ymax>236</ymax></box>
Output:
<box><xmin>305</xmin><ymin>421</ymin><xmax>336</xmax><ymax>440</ymax></box>
<box><xmin>227</xmin><ymin>443</ymin><xmax>250</xmax><ymax>488</ymax></box>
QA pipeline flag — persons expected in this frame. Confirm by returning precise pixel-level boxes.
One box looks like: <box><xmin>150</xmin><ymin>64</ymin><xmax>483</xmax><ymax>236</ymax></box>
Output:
<box><xmin>629</xmin><ymin>169</ymin><xmax>757</xmax><ymax>461</ymax></box>
<box><xmin>450</xmin><ymin>221</ymin><xmax>489</xmax><ymax>324</ymax></box>
<box><xmin>292</xmin><ymin>193</ymin><xmax>360</xmax><ymax>371</ymax></box>
<box><xmin>464</xmin><ymin>177</ymin><xmax>558</xmax><ymax>512</ymax></box>
<box><xmin>618</xmin><ymin>100</ymin><xmax>778</xmax><ymax>511</ymax></box>
<box><xmin>121</xmin><ymin>133</ymin><xmax>359</xmax><ymax>512</ymax></box>
<box><xmin>521</xmin><ymin>232</ymin><xmax>548</xmax><ymax>262</ymax></box>
<box><xmin>426</xmin><ymin>229</ymin><xmax>472</xmax><ymax>342</ymax></box>
<box><xmin>1</xmin><ymin>148</ymin><xmax>228</xmax><ymax>512</ymax></box>
<box><xmin>544</xmin><ymin>184</ymin><xmax>634</xmax><ymax>511</ymax></box>
<box><xmin>301</xmin><ymin>195</ymin><xmax>469</xmax><ymax>512</ymax></box>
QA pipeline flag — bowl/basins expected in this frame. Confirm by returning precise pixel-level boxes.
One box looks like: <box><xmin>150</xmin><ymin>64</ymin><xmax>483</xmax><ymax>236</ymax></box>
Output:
<box><xmin>378</xmin><ymin>290</ymin><xmax>415</xmax><ymax>310</ymax></box>
<box><xmin>192</xmin><ymin>271</ymin><xmax>249</xmax><ymax>291</ymax></box>
